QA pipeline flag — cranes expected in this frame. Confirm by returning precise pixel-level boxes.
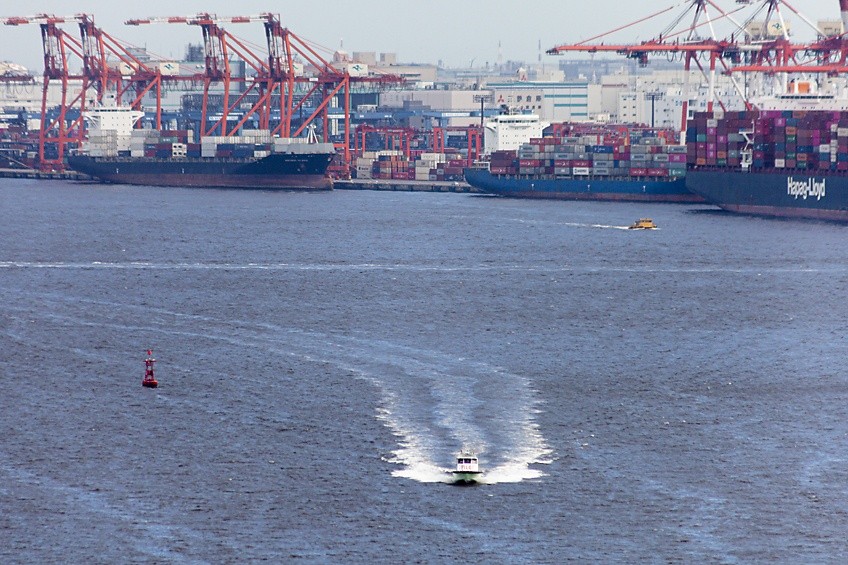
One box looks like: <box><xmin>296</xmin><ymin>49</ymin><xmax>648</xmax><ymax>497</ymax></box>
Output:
<box><xmin>0</xmin><ymin>13</ymin><xmax>163</xmax><ymax>162</ymax></box>
<box><xmin>122</xmin><ymin>13</ymin><xmax>350</xmax><ymax>164</ymax></box>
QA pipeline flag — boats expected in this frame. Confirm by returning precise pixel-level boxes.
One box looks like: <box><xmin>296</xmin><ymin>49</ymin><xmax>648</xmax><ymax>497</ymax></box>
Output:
<box><xmin>450</xmin><ymin>451</ymin><xmax>483</xmax><ymax>485</ymax></box>
<box><xmin>464</xmin><ymin>135</ymin><xmax>711</xmax><ymax>203</ymax></box>
<box><xmin>629</xmin><ymin>218</ymin><xmax>655</xmax><ymax>229</ymax></box>
<box><xmin>65</xmin><ymin>91</ymin><xmax>339</xmax><ymax>191</ymax></box>
<box><xmin>685</xmin><ymin>110</ymin><xmax>848</xmax><ymax>222</ymax></box>
